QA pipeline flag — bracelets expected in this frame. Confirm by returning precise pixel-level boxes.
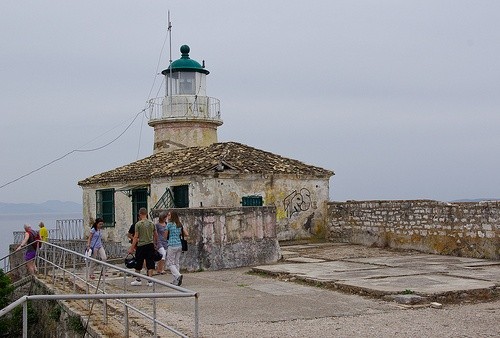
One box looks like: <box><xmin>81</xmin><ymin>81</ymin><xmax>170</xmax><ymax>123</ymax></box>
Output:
<box><xmin>20</xmin><ymin>245</ymin><xmax>23</xmax><ymax>248</ymax></box>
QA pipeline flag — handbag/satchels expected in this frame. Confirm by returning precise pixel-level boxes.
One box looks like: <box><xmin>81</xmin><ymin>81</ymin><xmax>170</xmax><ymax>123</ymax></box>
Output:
<box><xmin>154</xmin><ymin>250</ymin><xmax>163</xmax><ymax>262</ymax></box>
<box><xmin>180</xmin><ymin>239</ymin><xmax>188</xmax><ymax>251</ymax></box>
<box><xmin>84</xmin><ymin>248</ymin><xmax>94</xmax><ymax>258</ymax></box>
<box><xmin>125</xmin><ymin>253</ymin><xmax>138</xmax><ymax>269</ymax></box>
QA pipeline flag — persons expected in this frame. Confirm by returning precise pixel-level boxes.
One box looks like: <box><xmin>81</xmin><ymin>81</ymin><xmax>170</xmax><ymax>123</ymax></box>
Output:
<box><xmin>16</xmin><ymin>224</ymin><xmax>38</xmax><ymax>277</ymax></box>
<box><xmin>87</xmin><ymin>218</ymin><xmax>108</xmax><ymax>279</ymax></box>
<box><xmin>155</xmin><ymin>211</ymin><xmax>168</xmax><ymax>274</ymax></box>
<box><xmin>128</xmin><ymin>208</ymin><xmax>157</xmax><ymax>286</ymax></box>
<box><xmin>128</xmin><ymin>213</ymin><xmax>148</xmax><ymax>277</ymax></box>
<box><xmin>164</xmin><ymin>211</ymin><xmax>188</xmax><ymax>286</ymax></box>
<box><xmin>38</xmin><ymin>222</ymin><xmax>49</xmax><ymax>266</ymax></box>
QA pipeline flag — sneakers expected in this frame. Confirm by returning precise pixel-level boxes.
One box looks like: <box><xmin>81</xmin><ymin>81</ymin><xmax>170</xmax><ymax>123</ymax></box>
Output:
<box><xmin>131</xmin><ymin>280</ymin><xmax>143</xmax><ymax>286</ymax></box>
<box><xmin>170</xmin><ymin>274</ymin><xmax>183</xmax><ymax>286</ymax></box>
<box><xmin>146</xmin><ymin>281</ymin><xmax>153</xmax><ymax>286</ymax></box>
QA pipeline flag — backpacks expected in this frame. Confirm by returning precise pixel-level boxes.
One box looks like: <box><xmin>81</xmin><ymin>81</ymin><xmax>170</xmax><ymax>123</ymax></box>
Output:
<box><xmin>27</xmin><ymin>230</ymin><xmax>41</xmax><ymax>248</ymax></box>
